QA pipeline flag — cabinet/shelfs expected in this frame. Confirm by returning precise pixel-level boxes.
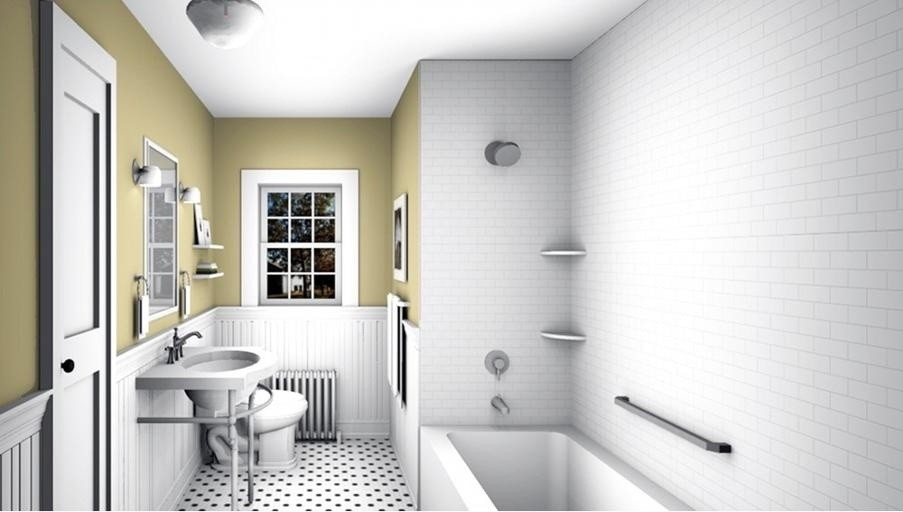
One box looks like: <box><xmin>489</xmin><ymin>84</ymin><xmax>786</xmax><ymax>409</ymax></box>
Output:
<box><xmin>192</xmin><ymin>243</ymin><xmax>225</xmax><ymax>280</ymax></box>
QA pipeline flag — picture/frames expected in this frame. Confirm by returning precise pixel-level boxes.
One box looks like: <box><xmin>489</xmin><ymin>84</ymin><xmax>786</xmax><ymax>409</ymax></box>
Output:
<box><xmin>392</xmin><ymin>191</ymin><xmax>407</xmax><ymax>284</ymax></box>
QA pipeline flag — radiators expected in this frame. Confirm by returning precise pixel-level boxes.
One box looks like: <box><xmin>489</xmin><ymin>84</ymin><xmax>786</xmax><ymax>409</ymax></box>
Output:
<box><xmin>270</xmin><ymin>369</ymin><xmax>341</xmax><ymax>446</ymax></box>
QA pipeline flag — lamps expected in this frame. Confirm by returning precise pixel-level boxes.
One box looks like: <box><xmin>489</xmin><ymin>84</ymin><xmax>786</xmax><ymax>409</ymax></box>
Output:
<box><xmin>132</xmin><ymin>159</ymin><xmax>162</xmax><ymax>188</ymax></box>
<box><xmin>178</xmin><ymin>182</ymin><xmax>201</xmax><ymax>204</ymax></box>
<box><xmin>185</xmin><ymin>0</ymin><xmax>267</xmax><ymax>52</ymax></box>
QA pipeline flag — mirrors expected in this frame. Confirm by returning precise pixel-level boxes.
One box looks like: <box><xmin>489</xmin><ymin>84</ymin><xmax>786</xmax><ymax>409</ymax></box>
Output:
<box><xmin>142</xmin><ymin>135</ymin><xmax>181</xmax><ymax>322</ymax></box>
